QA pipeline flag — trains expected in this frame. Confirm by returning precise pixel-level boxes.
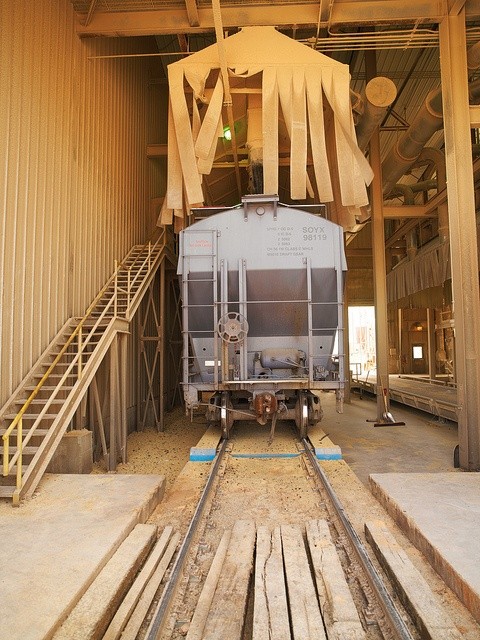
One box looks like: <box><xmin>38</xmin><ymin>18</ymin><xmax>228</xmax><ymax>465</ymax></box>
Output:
<box><xmin>176</xmin><ymin>194</ymin><xmax>349</xmax><ymax>441</ymax></box>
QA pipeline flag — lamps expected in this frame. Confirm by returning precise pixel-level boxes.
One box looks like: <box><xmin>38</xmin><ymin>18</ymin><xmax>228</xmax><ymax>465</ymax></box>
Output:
<box><xmin>415</xmin><ymin>322</ymin><xmax>422</xmax><ymax>330</ymax></box>
<box><xmin>224</xmin><ymin>129</ymin><xmax>234</xmax><ymax>141</ymax></box>
